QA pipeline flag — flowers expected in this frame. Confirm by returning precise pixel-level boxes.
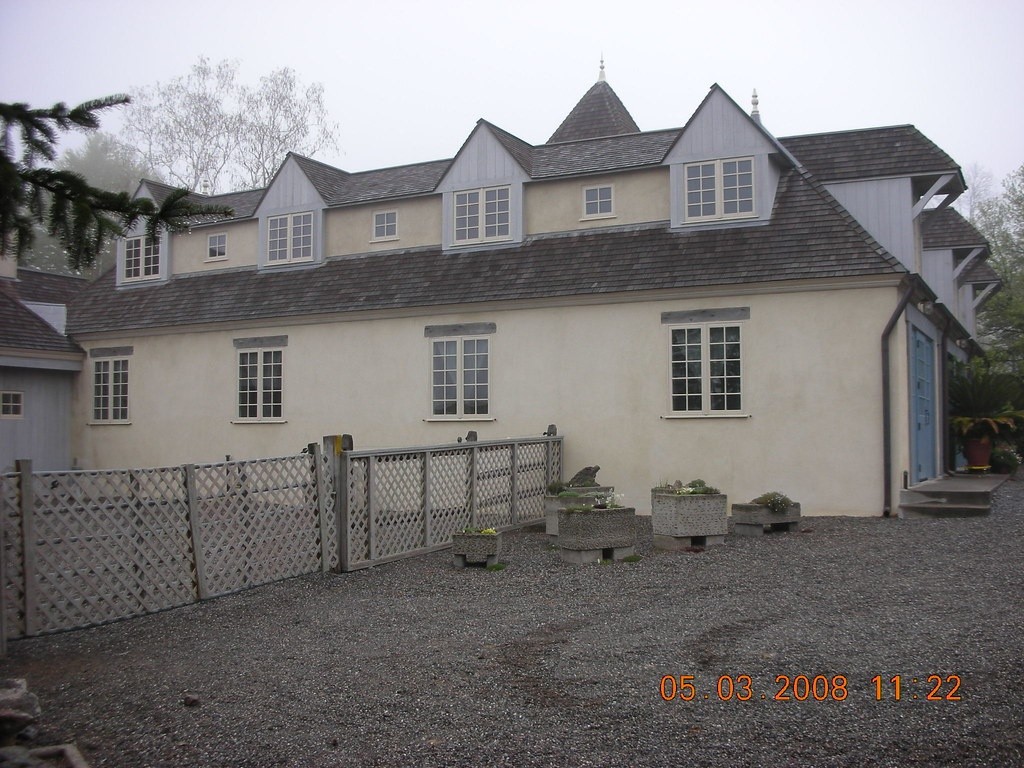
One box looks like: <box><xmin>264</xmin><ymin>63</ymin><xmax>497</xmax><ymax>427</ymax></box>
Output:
<box><xmin>651</xmin><ymin>477</ymin><xmax>720</xmax><ymax>495</ymax></box>
<box><xmin>755</xmin><ymin>491</ymin><xmax>795</xmax><ymax>513</ymax></box>
<box><xmin>594</xmin><ymin>488</ymin><xmax>626</xmax><ymax>509</ymax></box>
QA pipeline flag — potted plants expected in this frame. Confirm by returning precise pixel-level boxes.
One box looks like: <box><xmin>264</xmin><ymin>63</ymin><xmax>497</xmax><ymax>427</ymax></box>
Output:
<box><xmin>545</xmin><ymin>481</ymin><xmax>614</xmax><ymax>536</ymax></box>
<box><xmin>452</xmin><ymin>527</ymin><xmax>503</xmax><ymax>555</ymax></box>
<box><xmin>942</xmin><ymin>370</ymin><xmax>1024</xmax><ymax>467</ymax></box>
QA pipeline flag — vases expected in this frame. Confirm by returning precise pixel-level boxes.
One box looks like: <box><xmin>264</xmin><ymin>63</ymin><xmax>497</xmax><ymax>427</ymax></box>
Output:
<box><xmin>557</xmin><ymin>507</ymin><xmax>636</xmax><ymax>550</ymax></box>
<box><xmin>731</xmin><ymin>502</ymin><xmax>801</xmax><ymax>525</ymax></box>
<box><xmin>651</xmin><ymin>487</ymin><xmax>728</xmax><ymax>536</ymax></box>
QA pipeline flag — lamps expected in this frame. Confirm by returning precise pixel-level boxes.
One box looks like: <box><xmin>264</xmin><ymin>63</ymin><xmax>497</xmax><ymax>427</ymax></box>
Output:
<box><xmin>917</xmin><ymin>300</ymin><xmax>934</xmax><ymax>315</ymax></box>
<box><xmin>956</xmin><ymin>339</ymin><xmax>967</xmax><ymax>349</ymax></box>
